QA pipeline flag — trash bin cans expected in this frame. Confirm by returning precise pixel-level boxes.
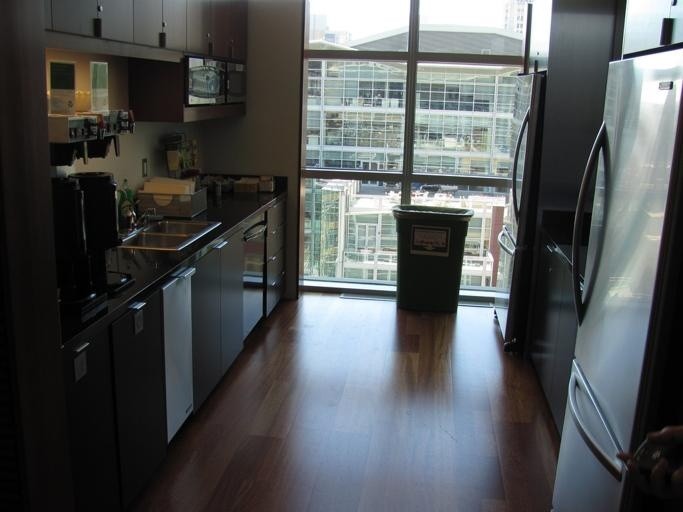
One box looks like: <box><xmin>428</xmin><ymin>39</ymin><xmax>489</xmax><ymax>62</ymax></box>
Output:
<box><xmin>392</xmin><ymin>204</ymin><xmax>474</xmax><ymax>313</ymax></box>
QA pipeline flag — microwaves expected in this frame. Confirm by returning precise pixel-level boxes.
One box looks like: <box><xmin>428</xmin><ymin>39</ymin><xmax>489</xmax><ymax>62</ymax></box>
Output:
<box><xmin>183</xmin><ymin>55</ymin><xmax>248</xmax><ymax>110</ymax></box>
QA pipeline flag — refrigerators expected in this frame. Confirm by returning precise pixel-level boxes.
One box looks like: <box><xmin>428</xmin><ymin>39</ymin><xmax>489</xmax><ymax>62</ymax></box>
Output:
<box><xmin>550</xmin><ymin>45</ymin><xmax>683</xmax><ymax>512</ymax></box>
<box><xmin>493</xmin><ymin>73</ymin><xmax>545</xmax><ymax>351</ymax></box>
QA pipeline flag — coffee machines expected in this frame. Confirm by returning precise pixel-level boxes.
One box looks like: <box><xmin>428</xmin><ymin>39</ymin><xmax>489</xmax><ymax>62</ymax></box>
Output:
<box><xmin>46</xmin><ymin>109</ymin><xmax>138</xmax><ymax>316</ymax></box>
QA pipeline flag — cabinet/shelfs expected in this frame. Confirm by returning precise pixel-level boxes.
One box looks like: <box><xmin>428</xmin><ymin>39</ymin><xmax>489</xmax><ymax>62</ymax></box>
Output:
<box><xmin>220</xmin><ymin>227</ymin><xmax>245</xmax><ymax>380</ymax></box>
<box><xmin>110</xmin><ymin>288</ymin><xmax>165</xmax><ymax>507</ymax></box>
<box><xmin>191</xmin><ymin>245</ymin><xmax>221</xmax><ymax>418</ymax></box>
<box><xmin>134</xmin><ymin>2</ymin><xmax>187</xmax><ymax>52</ymax></box>
<box><xmin>267</xmin><ymin>198</ymin><xmax>285</xmax><ymax>318</ymax></box>
<box><xmin>43</xmin><ymin>2</ymin><xmax>134</xmax><ymax>45</ymax></box>
<box><xmin>186</xmin><ymin>1</ymin><xmax>248</xmax><ymax>62</ymax></box>
<box><xmin>64</xmin><ymin>326</ymin><xmax>121</xmax><ymax>510</ymax></box>
<box><xmin>162</xmin><ymin>265</ymin><xmax>196</xmax><ymax>448</ymax></box>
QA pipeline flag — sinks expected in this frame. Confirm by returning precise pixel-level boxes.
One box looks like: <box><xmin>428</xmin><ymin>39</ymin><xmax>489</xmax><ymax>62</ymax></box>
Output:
<box><xmin>141</xmin><ymin>219</ymin><xmax>222</xmax><ymax>237</ymax></box>
<box><xmin>114</xmin><ymin>231</ymin><xmax>195</xmax><ymax>252</ymax></box>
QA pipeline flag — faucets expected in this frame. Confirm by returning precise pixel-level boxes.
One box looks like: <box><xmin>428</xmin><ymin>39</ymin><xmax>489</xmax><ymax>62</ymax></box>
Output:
<box><xmin>129</xmin><ymin>199</ymin><xmax>156</xmax><ymax>231</ymax></box>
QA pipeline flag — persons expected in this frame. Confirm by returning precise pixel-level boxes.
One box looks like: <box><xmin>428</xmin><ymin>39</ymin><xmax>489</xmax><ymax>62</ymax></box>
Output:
<box><xmin>615</xmin><ymin>424</ymin><xmax>683</xmax><ymax>502</ymax></box>
